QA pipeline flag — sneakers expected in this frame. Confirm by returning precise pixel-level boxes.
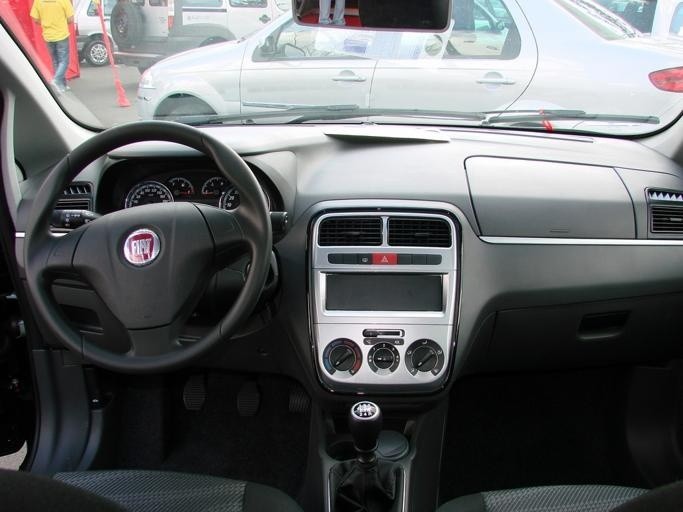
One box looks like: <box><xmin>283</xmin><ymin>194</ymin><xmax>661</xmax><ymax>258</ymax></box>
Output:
<box><xmin>50</xmin><ymin>80</ymin><xmax>71</xmax><ymax>94</ymax></box>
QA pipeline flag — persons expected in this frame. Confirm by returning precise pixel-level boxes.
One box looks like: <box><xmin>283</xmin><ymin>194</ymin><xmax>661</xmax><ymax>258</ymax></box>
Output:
<box><xmin>319</xmin><ymin>0</ymin><xmax>346</xmax><ymax>26</ymax></box>
<box><xmin>30</xmin><ymin>0</ymin><xmax>74</xmax><ymax>93</ymax></box>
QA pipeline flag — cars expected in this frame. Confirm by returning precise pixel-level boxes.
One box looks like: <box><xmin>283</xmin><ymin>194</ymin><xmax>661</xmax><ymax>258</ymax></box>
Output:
<box><xmin>136</xmin><ymin>2</ymin><xmax>683</xmax><ymax>158</ymax></box>
<box><xmin>74</xmin><ymin>0</ymin><xmax>117</xmax><ymax>64</ymax></box>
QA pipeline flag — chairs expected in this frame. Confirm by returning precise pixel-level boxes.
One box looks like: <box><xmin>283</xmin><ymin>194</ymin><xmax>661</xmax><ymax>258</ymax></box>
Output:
<box><xmin>3</xmin><ymin>470</ymin><xmax>308</xmax><ymax>512</ymax></box>
<box><xmin>431</xmin><ymin>480</ymin><xmax>681</xmax><ymax>511</ymax></box>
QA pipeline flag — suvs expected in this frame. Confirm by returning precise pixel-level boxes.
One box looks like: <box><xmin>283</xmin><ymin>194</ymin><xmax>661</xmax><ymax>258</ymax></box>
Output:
<box><xmin>112</xmin><ymin>2</ymin><xmax>285</xmax><ymax>70</ymax></box>
